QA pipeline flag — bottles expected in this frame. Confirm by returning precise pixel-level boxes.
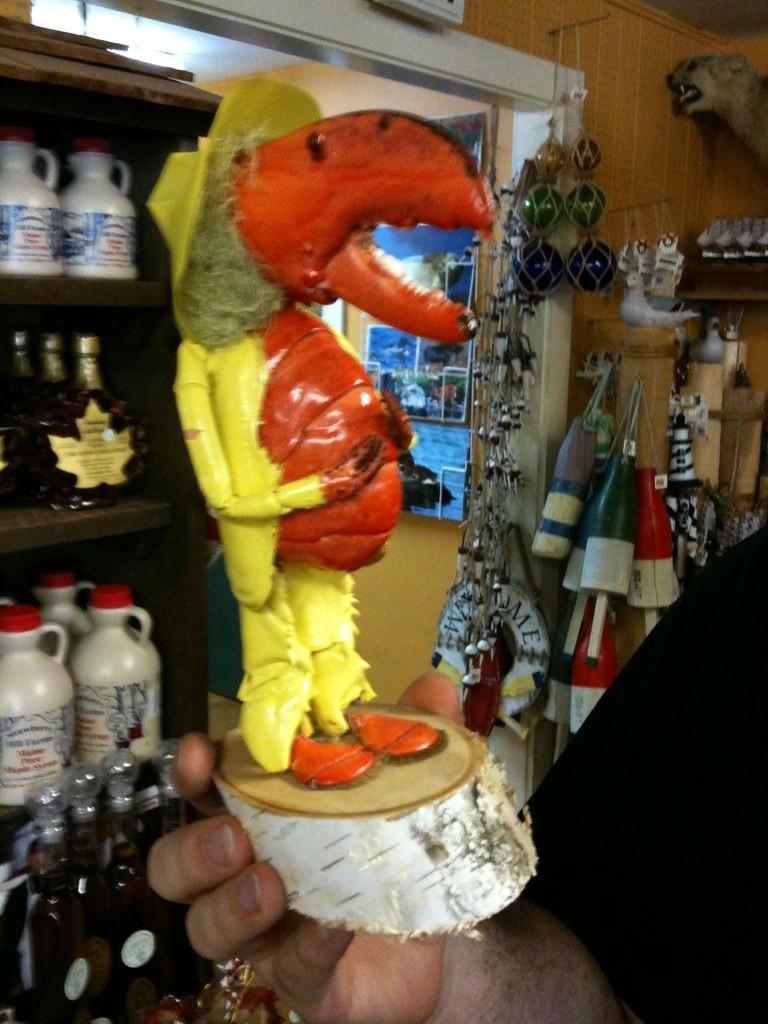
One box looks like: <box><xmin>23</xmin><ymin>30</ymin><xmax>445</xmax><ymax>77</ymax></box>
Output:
<box><xmin>0</xmin><ymin>738</ymin><xmax>212</xmax><ymax>1024</ymax></box>
<box><xmin>60</xmin><ymin>133</ymin><xmax>138</xmax><ymax>279</ymax></box>
<box><xmin>0</xmin><ymin>571</ymin><xmax>160</xmax><ymax>807</ymax></box>
<box><xmin>2</xmin><ymin>327</ymin><xmax>148</xmax><ymax>508</ymax></box>
<box><xmin>1</xmin><ymin>125</ymin><xmax>64</xmax><ymax>276</ymax></box>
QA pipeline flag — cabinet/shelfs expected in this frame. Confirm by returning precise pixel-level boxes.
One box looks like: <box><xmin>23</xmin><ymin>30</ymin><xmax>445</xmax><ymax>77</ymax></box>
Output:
<box><xmin>1</xmin><ymin>18</ymin><xmax>209</xmax><ymax>832</ymax></box>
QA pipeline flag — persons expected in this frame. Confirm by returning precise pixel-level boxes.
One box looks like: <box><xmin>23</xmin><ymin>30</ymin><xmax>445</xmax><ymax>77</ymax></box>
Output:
<box><xmin>148</xmin><ymin>528</ymin><xmax>767</xmax><ymax>1023</ymax></box>
